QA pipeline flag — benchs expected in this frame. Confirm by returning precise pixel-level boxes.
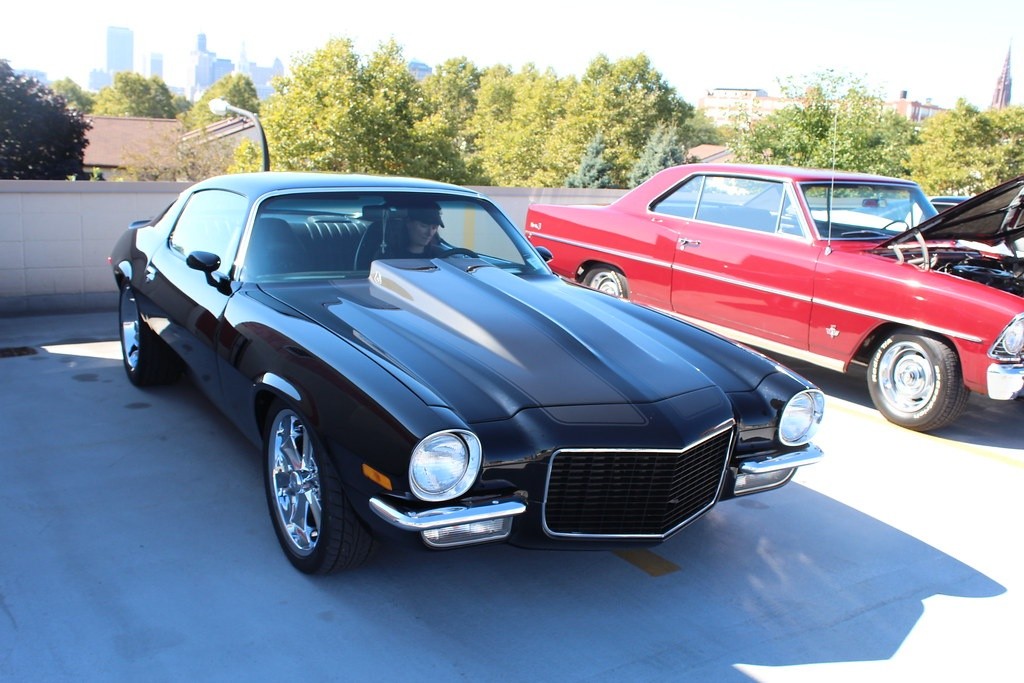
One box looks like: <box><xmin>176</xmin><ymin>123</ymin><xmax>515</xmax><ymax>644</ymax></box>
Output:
<box><xmin>290</xmin><ymin>220</ymin><xmax>378</xmax><ymax>270</ymax></box>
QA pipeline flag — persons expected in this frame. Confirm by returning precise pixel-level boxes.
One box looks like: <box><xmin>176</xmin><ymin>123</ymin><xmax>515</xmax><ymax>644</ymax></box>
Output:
<box><xmin>371</xmin><ymin>199</ymin><xmax>447</xmax><ymax>259</ymax></box>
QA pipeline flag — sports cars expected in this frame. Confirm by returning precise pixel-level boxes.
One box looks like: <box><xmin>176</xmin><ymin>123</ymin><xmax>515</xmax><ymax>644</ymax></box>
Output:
<box><xmin>523</xmin><ymin>161</ymin><xmax>1024</xmax><ymax>432</ymax></box>
<box><xmin>108</xmin><ymin>170</ymin><xmax>828</xmax><ymax>577</ymax></box>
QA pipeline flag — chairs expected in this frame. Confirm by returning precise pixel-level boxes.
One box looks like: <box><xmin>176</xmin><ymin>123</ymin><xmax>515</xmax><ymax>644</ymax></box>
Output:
<box><xmin>353</xmin><ymin>214</ymin><xmax>411</xmax><ymax>271</ymax></box>
<box><xmin>719</xmin><ymin>204</ymin><xmax>766</xmax><ymax>233</ymax></box>
<box><xmin>244</xmin><ymin>215</ymin><xmax>312</xmax><ymax>273</ymax></box>
